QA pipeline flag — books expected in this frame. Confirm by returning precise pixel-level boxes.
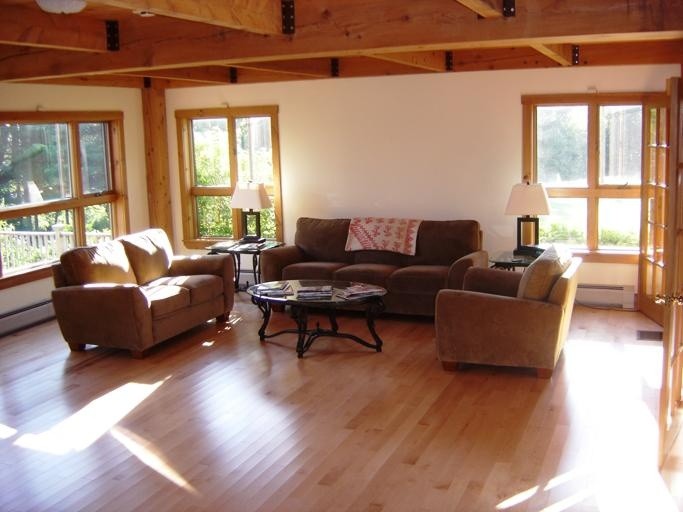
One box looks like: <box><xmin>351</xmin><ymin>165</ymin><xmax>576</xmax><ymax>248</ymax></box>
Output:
<box><xmin>237</xmin><ymin>241</ymin><xmax>264</xmax><ymax>249</ymax></box>
<box><xmin>257</xmin><ymin>279</ymin><xmax>383</xmax><ymax>298</ymax></box>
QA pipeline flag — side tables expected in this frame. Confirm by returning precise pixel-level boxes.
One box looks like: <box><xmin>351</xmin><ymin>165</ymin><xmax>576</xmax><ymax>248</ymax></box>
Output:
<box><xmin>204</xmin><ymin>239</ymin><xmax>286</xmax><ymax>293</ymax></box>
<box><xmin>487</xmin><ymin>250</ymin><xmax>534</xmax><ymax>271</ymax></box>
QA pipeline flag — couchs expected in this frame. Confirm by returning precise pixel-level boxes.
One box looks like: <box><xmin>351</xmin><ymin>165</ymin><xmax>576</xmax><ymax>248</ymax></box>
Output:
<box><xmin>259</xmin><ymin>216</ymin><xmax>487</xmax><ymax>316</ymax></box>
<box><xmin>50</xmin><ymin>228</ymin><xmax>238</xmax><ymax>359</ymax></box>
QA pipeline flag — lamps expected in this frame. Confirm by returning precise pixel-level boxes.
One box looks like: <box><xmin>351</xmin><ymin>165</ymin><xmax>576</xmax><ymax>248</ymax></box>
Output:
<box><xmin>229</xmin><ymin>178</ymin><xmax>272</xmax><ymax>244</ymax></box>
<box><xmin>503</xmin><ymin>180</ymin><xmax>550</xmax><ymax>255</ymax></box>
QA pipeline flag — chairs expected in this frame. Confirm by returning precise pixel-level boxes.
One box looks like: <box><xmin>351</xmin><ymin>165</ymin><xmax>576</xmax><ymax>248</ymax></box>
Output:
<box><xmin>433</xmin><ymin>243</ymin><xmax>584</xmax><ymax>379</ymax></box>
